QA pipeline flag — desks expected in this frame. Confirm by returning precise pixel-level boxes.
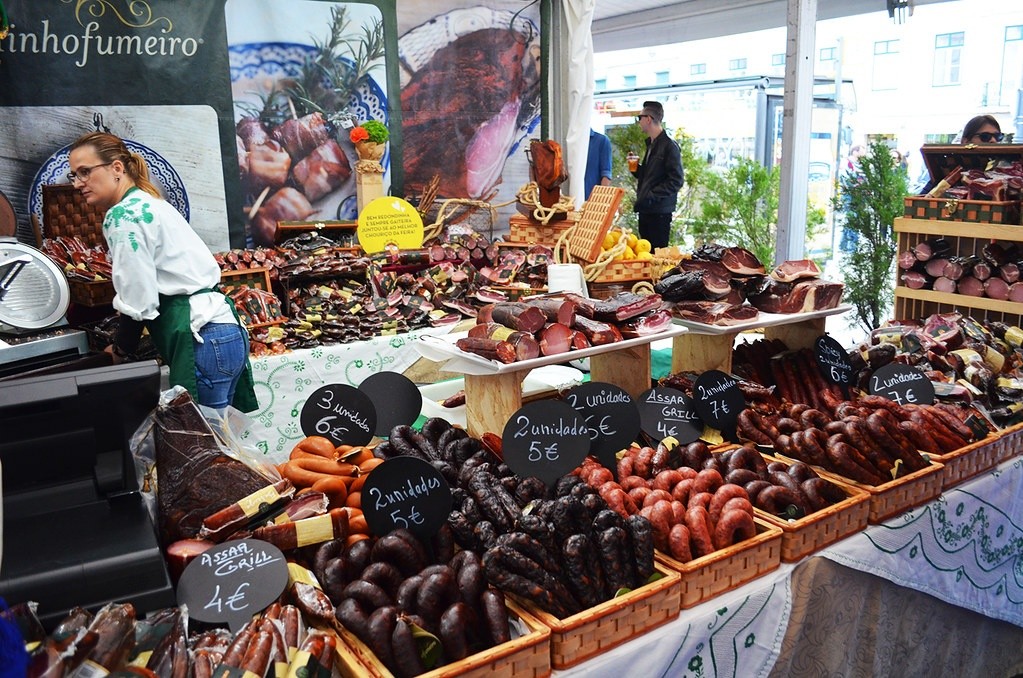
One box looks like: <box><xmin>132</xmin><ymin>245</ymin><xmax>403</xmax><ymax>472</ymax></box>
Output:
<box><xmin>228</xmin><ymin>318</ymin><xmax>477</xmax><ymax>467</ymax></box>
<box><xmin>671</xmin><ymin>302</ymin><xmax>853</xmax><ymax>376</ymax></box>
<box><xmin>411</xmin><ymin>324</ymin><xmax>689</xmax><ymax>441</ymax></box>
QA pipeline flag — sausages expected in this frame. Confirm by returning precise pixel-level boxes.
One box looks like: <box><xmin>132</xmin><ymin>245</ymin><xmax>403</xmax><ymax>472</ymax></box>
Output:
<box><xmin>260</xmin><ymin>435</ymin><xmax>511</xmax><ymax>677</ymax></box>
<box><xmin>454</xmin><ymin>298</ymin><xmax>578</xmax><ymax>363</ymax></box>
<box><xmin>35</xmin><ymin>235</ymin><xmax>557</xmax><ymax>355</ymax></box>
<box><xmin>567</xmin><ymin>438</ymin><xmax>847</xmax><ymax>561</ymax></box>
<box><xmin>897</xmin><ymin>239</ymin><xmax>1023</xmax><ymax>303</ymax></box>
<box><xmin>373</xmin><ymin>415</ymin><xmax>657</xmax><ymax>617</ymax></box>
<box><xmin>441</xmin><ymin>390</ymin><xmax>465</xmax><ymax>408</ymax></box>
<box><xmin>185</xmin><ymin>602</ymin><xmax>337</xmax><ymax>678</ymax></box>
<box><xmin>731</xmin><ymin>337</ymin><xmax>977</xmax><ymax>484</ymax></box>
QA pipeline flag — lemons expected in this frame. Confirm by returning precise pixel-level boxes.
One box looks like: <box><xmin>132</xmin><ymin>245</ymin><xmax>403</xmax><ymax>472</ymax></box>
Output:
<box><xmin>594</xmin><ymin>230</ymin><xmax>655</xmax><ymax>259</ymax></box>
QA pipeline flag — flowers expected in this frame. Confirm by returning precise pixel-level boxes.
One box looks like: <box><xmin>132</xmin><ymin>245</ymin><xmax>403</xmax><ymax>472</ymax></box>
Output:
<box><xmin>348</xmin><ymin>120</ymin><xmax>391</xmax><ymax>144</ymax></box>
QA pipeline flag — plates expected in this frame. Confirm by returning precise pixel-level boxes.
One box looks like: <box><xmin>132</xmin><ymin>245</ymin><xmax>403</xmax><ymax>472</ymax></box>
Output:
<box><xmin>1</xmin><ymin>192</ymin><xmax>16</xmax><ymax>237</ymax></box>
<box><xmin>227</xmin><ymin>42</ymin><xmax>392</xmax><ymax>221</ymax></box>
<box><xmin>27</xmin><ymin>138</ymin><xmax>189</xmax><ymax>246</ymax></box>
<box><xmin>0</xmin><ymin>241</ymin><xmax>70</xmax><ymax>328</ymax></box>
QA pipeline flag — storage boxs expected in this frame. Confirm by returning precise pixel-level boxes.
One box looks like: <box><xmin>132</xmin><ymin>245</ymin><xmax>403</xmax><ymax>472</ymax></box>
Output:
<box><xmin>417</xmin><ymin>376</ymin><xmax>555</xmax><ymax>430</ymax></box>
<box><xmin>273</xmin><ymin>220</ymin><xmax>360</xmax><ymax>256</ymax></box>
<box><xmin>904</xmin><ymin>142</ymin><xmax>1023</xmax><ymax>226</ymax></box>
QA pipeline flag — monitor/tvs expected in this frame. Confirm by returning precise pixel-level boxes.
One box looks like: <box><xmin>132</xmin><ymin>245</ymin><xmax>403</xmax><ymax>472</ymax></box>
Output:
<box><xmin>0</xmin><ymin>359</ymin><xmax>176</xmax><ymax>630</ymax></box>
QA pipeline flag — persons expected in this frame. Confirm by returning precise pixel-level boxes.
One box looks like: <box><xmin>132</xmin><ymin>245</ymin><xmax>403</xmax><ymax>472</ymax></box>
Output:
<box><xmin>65</xmin><ymin>132</ymin><xmax>250</xmax><ymax>435</ymax></box>
<box><xmin>838</xmin><ymin>144</ymin><xmax>903</xmax><ymax>254</ymax></box>
<box><xmin>627</xmin><ymin>101</ymin><xmax>685</xmax><ymax>254</ymax></box>
<box><xmin>961</xmin><ymin>115</ymin><xmax>1002</xmax><ymax>144</ymax></box>
<box><xmin>584</xmin><ymin>128</ymin><xmax>612</xmax><ymax>201</ymax></box>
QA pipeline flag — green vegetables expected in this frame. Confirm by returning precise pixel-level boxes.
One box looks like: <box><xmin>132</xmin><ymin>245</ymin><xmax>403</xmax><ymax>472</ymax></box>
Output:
<box><xmin>231</xmin><ymin>3</ymin><xmax>383</xmax><ymax>140</ymax></box>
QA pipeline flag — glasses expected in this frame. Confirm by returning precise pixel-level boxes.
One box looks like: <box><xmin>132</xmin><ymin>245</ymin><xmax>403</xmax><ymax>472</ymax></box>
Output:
<box><xmin>638</xmin><ymin>114</ymin><xmax>655</xmax><ymax>121</ymax></box>
<box><xmin>974</xmin><ymin>132</ymin><xmax>1005</xmax><ymax>143</ymax></box>
<box><xmin>67</xmin><ymin>163</ymin><xmax>113</xmax><ymax>185</ymax></box>
